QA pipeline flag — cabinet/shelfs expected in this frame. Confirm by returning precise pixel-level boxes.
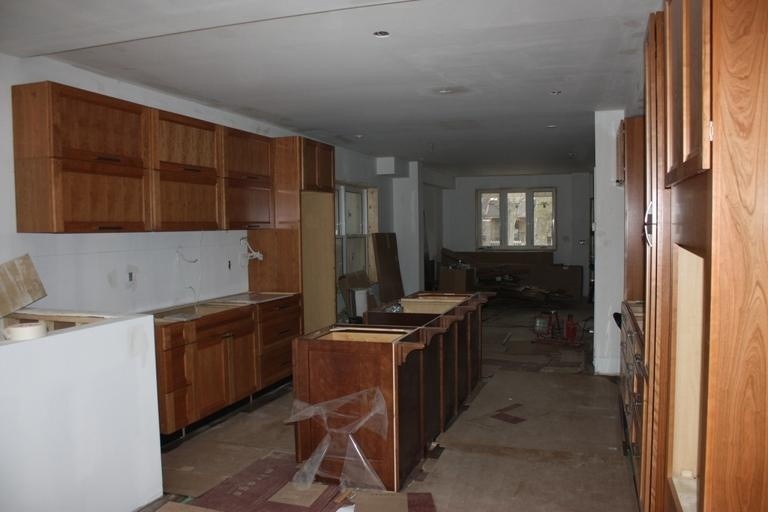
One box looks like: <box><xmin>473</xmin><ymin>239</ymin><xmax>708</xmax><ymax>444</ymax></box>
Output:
<box><xmin>291</xmin><ymin>291</ymin><xmax>498</xmax><ymax>494</ymax></box>
<box><xmin>13</xmin><ymin>158</ymin><xmax>151</xmax><ymax>233</ymax></box>
<box><xmin>13</xmin><ymin>83</ymin><xmax>149</xmax><ymax>169</ymax></box>
<box><xmin>615</xmin><ymin>0</ymin><xmax>768</xmax><ymax>512</ymax></box>
<box><xmin>153</xmin><ymin>324</ymin><xmax>195</xmax><ymax>435</ymax></box>
<box><xmin>299</xmin><ymin>138</ymin><xmax>334</xmax><ymax>190</ymax></box>
<box><xmin>259</xmin><ymin>310</ymin><xmax>301</xmax><ymax>392</ymax></box>
<box><xmin>222</xmin><ymin>181</ymin><xmax>277</xmax><ymax>230</ymax></box>
<box><xmin>221</xmin><ymin>126</ymin><xmax>274</xmax><ymax>184</ymax></box>
<box><xmin>151</xmin><ymin>171</ymin><xmax>222</xmax><ymax>230</ymax></box>
<box><xmin>198</xmin><ymin>318</ymin><xmax>262</xmax><ymax>417</ymax></box>
<box><xmin>151</xmin><ymin>111</ymin><xmax>219</xmax><ymax>180</ymax></box>
<box><xmin>298</xmin><ymin>193</ymin><xmax>340</xmax><ymax>339</ymax></box>
